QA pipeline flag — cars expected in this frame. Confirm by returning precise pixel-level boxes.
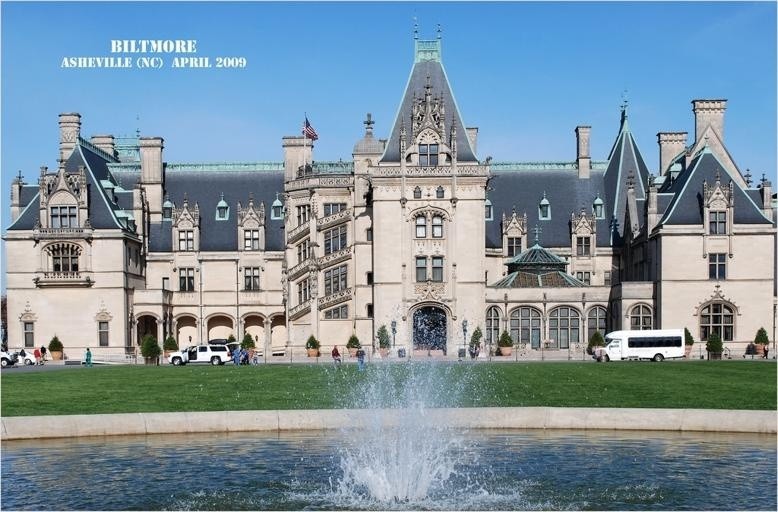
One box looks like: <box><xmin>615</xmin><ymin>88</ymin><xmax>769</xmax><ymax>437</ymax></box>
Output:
<box><xmin>0</xmin><ymin>345</ymin><xmax>42</xmax><ymax>368</ymax></box>
<box><xmin>167</xmin><ymin>339</ymin><xmax>240</xmax><ymax>366</ymax></box>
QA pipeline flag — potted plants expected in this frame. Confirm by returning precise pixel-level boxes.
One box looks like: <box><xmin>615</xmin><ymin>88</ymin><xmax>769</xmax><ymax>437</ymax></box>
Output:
<box><xmin>685</xmin><ymin>328</ymin><xmax>723</xmax><ymax>360</ymax></box>
<box><xmin>241</xmin><ymin>333</ymin><xmax>256</xmax><ymax>358</ymax></box>
<box><xmin>305</xmin><ymin>335</ymin><xmax>321</xmax><ymax>356</ymax></box>
<box><xmin>377</xmin><ymin>326</ymin><xmax>390</xmax><ymax>356</ymax></box>
<box><xmin>754</xmin><ymin>328</ymin><xmax>769</xmax><ymax>354</ymax></box>
<box><xmin>498</xmin><ymin>330</ymin><xmax>514</xmax><ymax>356</ymax></box>
<box><xmin>589</xmin><ymin>331</ymin><xmax>604</xmax><ymax>354</ymax></box>
<box><xmin>139</xmin><ymin>333</ymin><xmax>178</xmax><ymax>366</ymax></box>
<box><xmin>48</xmin><ymin>336</ymin><xmax>63</xmax><ymax>360</ymax></box>
<box><xmin>346</xmin><ymin>335</ymin><xmax>360</xmax><ymax>357</ymax></box>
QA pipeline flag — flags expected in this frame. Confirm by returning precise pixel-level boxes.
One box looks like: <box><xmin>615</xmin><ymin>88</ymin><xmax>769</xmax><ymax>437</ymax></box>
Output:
<box><xmin>302</xmin><ymin>118</ymin><xmax>318</xmax><ymax>141</ymax></box>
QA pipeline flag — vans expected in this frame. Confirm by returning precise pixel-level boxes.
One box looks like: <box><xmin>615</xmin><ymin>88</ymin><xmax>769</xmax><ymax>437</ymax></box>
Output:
<box><xmin>592</xmin><ymin>328</ymin><xmax>686</xmax><ymax>363</ymax></box>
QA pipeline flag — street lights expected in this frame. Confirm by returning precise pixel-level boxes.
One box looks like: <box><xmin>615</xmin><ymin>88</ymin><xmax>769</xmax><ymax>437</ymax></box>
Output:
<box><xmin>462</xmin><ymin>320</ymin><xmax>467</xmax><ymax>348</ymax></box>
<box><xmin>391</xmin><ymin>322</ymin><xmax>397</xmax><ymax>348</ymax></box>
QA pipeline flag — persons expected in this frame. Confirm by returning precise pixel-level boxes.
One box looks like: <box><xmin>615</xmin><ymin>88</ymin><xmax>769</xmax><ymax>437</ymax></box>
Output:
<box><xmin>252</xmin><ymin>351</ymin><xmax>259</xmax><ymax>366</ymax></box>
<box><xmin>761</xmin><ymin>343</ymin><xmax>769</xmax><ymax>359</ymax></box>
<box><xmin>39</xmin><ymin>344</ymin><xmax>46</xmax><ymax>365</ymax></box>
<box><xmin>331</xmin><ymin>345</ymin><xmax>342</xmax><ymax>369</ymax></box>
<box><xmin>20</xmin><ymin>349</ymin><xmax>26</xmax><ymax>363</ymax></box>
<box><xmin>355</xmin><ymin>346</ymin><xmax>366</xmax><ymax>365</ymax></box>
<box><xmin>232</xmin><ymin>347</ymin><xmax>241</xmax><ymax>366</ymax></box>
<box><xmin>85</xmin><ymin>347</ymin><xmax>93</xmax><ymax>368</ymax></box>
<box><xmin>239</xmin><ymin>349</ymin><xmax>249</xmax><ymax>365</ymax></box>
<box><xmin>34</xmin><ymin>348</ymin><xmax>40</xmax><ymax>365</ymax></box>
